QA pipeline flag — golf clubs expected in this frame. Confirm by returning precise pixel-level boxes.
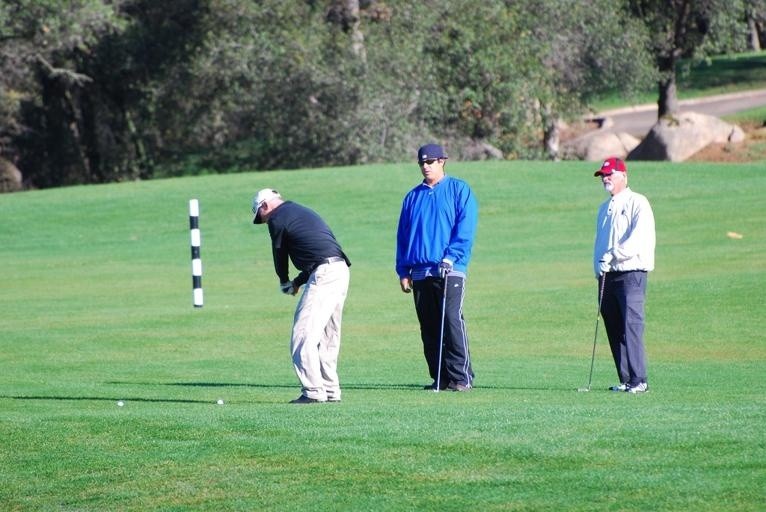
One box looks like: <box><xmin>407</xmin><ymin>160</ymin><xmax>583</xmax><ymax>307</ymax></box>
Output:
<box><xmin>578</xmin><ymin>270</ymin><xmax>607</xmax><ymax>393</ymax></box>
<box><xmin>429</xmin><ymin>271</ymin><xmax>449</xmax><ymax>393</ymax></box>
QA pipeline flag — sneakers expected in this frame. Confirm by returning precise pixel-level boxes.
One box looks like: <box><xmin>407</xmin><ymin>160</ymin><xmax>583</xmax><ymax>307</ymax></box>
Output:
<box><xmin>424</xmin><ymin>379</ymin><xmax>456</xmax><ymax>391</ymax></box>
<box><xmin>452</xmin><ymin>383</ymin><xmax>472</xmax><ymax>391</ymax></box>
<box><xmin>609</xmin><ymin>381</ymin><xmax>649</xmax><ymax>394</ymax></box>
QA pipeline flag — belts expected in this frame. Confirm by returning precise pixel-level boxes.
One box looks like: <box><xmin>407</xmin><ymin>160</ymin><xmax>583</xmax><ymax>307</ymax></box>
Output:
<box><xmin>308</xmin><ymin>257</ymin><xmax>345</xmax><ymax>274</ymax></box>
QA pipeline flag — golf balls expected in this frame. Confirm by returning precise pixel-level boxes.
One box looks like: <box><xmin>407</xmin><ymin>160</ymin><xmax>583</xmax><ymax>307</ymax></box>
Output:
<box><xmin>117</xmin><ymin>400</ymin><xmax>125</xmax><ymax>407</ymax></box>
<box><xmin>217</xmin><ymin>399</ymin><xmax>224</xmax><ymax>406</ymax></box>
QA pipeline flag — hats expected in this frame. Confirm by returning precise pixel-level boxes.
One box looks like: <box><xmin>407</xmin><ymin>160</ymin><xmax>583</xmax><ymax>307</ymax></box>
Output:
<box><xmin>594</xmin><ymin>158</ymin><xmax>626</xmax><ymax>177</ymax></box>
<box><xmin>418</xmin><ymin>144</ymin><xmax>448</xmax><ymax>161</ymax></box>
<box><xmin>252</xmin><ymin>188</ymin><xmax>279</xmax><ymax>224</ymax></box>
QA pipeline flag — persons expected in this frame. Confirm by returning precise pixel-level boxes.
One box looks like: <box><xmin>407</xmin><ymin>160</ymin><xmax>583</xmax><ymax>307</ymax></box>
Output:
<box><xmin>594</xmin><ymin>158</ymin><xmax>655</xmax><ymax>394</ymax></box>
<box><xmin>395</xmin><ymin>143</ymin><xmax>478</xmax><ymax>392</ymax></box>
<box><xmin>252</xmin><ymin>188</ymin><xmax>351</xmax><ymax>404</ymax></box>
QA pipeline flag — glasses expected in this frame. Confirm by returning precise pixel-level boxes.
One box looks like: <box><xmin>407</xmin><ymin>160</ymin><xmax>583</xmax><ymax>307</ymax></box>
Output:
<box><xmin>418</xmin><ymin>159</ymin><xmax>438</xmax><ymax>167</ymax></box>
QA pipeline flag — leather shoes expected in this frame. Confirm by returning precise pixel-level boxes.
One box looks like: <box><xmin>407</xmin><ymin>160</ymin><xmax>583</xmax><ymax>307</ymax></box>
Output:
<box><xmin>288</xmin><ymin>394</ymin><xmax>341</xmax><ymax>403</ymax></box>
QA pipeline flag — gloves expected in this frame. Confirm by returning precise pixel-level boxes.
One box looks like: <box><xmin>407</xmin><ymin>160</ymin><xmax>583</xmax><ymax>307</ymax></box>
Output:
<box><xmin>438</xmin><ymin>258</ymin><xmax>453</xmax><ymax>279</ymax></box>
<box><xmin>280</xmin><ymin>280</ymin><xmax>294</xmax><ymax>294</ymax></box>
<box><xmin>598</xmin><ymin>252</ymin><xmax>616</xmax><ymax>277</ymax></box>
